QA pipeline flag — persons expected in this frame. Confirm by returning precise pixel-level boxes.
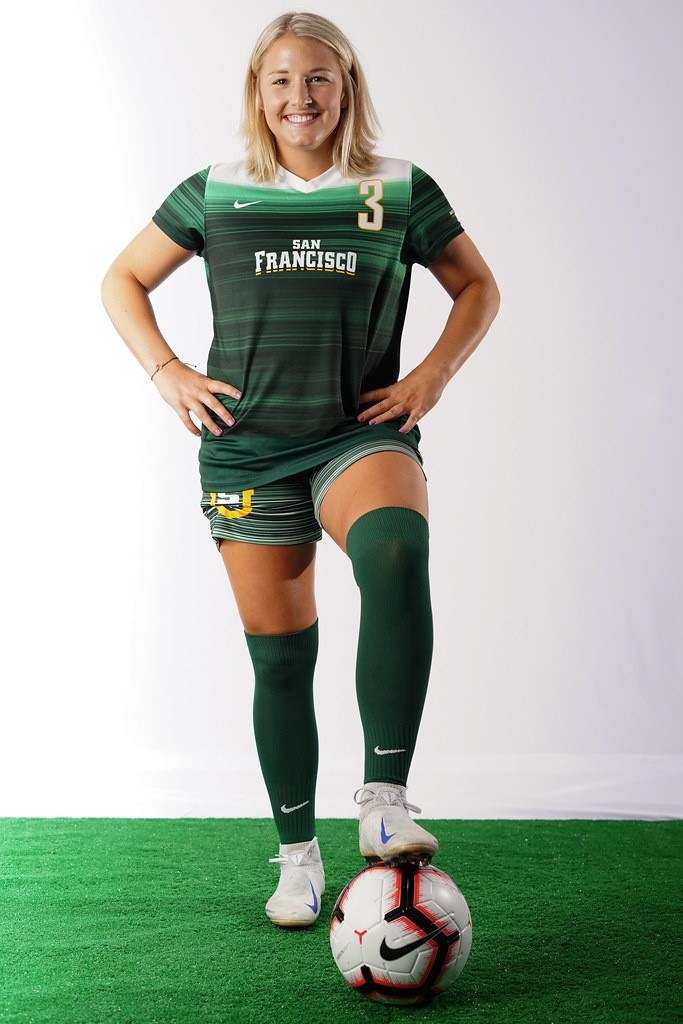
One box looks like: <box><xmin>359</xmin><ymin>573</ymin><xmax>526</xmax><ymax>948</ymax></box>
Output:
<box><xmin>99</xmin><ymin>10</ymin><xmax>502</xmax><ymax>930</ymax></box>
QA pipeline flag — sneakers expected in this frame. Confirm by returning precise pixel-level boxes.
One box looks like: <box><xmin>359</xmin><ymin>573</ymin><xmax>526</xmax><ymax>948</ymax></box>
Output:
<box><xmin>264</xmin><ymin>836</ymin><xmax>326</xmax><ymax>926</ymax></box>
<box><xmin>354</xmin><ymin>786</ymin><xmax>439</xmax><ymax>869</ymax></box>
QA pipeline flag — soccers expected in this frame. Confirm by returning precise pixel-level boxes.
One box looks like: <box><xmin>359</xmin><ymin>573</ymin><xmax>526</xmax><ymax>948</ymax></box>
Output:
<box><xmin>330</xmin><ymin>861</ymin><xmax>472</xmax><ymax>1006</ymax></box>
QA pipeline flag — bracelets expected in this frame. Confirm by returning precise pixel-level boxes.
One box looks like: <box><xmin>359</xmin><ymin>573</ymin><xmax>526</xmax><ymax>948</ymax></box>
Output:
<box><xmin>151</xmin><ymin>357</ymin><xmax>196</xmax><ymax>382</ymax></box>
